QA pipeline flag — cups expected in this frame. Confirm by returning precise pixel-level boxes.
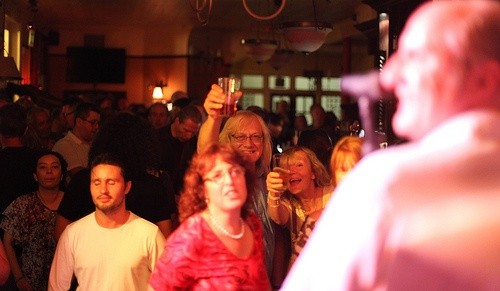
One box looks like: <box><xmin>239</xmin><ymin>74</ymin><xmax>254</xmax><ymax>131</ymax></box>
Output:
<box><xmin>272</xmin><ymin>153</ymin><xmax>289</xmax><ymax>189</ymax></box>
<box><xmin>218</xmin><ymin>77</ymin><xmax>240</xmax><ymax>116</ymax></box>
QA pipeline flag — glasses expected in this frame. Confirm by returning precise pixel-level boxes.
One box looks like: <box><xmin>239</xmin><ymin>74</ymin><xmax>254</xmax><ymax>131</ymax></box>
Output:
<box><xmin>202</xmin><ymin>167</ymin><xmax>246</xmax><ymax>183</ymax></box>
<box><xmin>80</xmin><ymin>117</ymin><xmax>101</xmax><ymax>126</ymax></box>
<box><xmin>231</xmin><ymin>134</ymin><xmax>264</xmax><ymax>143</ymax></box>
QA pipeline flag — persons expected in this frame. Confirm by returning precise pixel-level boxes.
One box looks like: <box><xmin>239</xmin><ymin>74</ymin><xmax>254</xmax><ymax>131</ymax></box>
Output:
<box><xmin>0</xmin><ymin>78</ymin><xmax>360</xmax><ymax>223</ymax></box>
<box><xmin>0</xmin><ymin>150</ymin><xmax>67</xmax><ymax>291</ymax></box>
<box><xmin>148</xmin><ymin>142</ymin><xmax>274</xmax><ymax>291</ymax></box>
<box><xmin>267</xmin><ymin>147</ymin><xmax>339</xmax><ymax>275</ymax></box>
<box><xmin>196</xmin><ymin>84</ymin><xmax>279</xmax><ymax>272</ymax></box>
<box><xmin>48</xmin><ymin>153</ymin><xmax>166</xmax><ymax>291</ymax></box>
<box><xmin>280</xmin><ymin>0</ymin><xmax>500</xmax><ymax>291</ymax></box>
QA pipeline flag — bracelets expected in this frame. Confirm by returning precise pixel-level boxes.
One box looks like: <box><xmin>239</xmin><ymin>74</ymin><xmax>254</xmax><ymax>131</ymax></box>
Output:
<box><xmin>15</xmin><ymin>275</ymin><xmax>25</xmax><ymax>283</ymax></box>
<box><xmin>268</xmin><ymin>192</ymin><xmax>280</xmax><ymax>200</ymax></box>
<box><xmin>266</xmin><ymin>200</ymin><xmax>281</xmax><ymax>209</ymax></box>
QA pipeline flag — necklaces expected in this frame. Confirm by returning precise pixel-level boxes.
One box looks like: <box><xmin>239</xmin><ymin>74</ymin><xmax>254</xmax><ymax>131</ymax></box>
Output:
<box><xmin>207</xmin><ymin>213</ymin><xmax>245</xmax><ymax>238</ymax></box>
<box><xmin>302</xmin><ymin>196</ymin><xmax>317</xmax><ymax>212</ymax></box>
<box><xmin>39</xmin><ymin>192</ymin><xmax>60</xmax><ymax>204</ymax></box>
